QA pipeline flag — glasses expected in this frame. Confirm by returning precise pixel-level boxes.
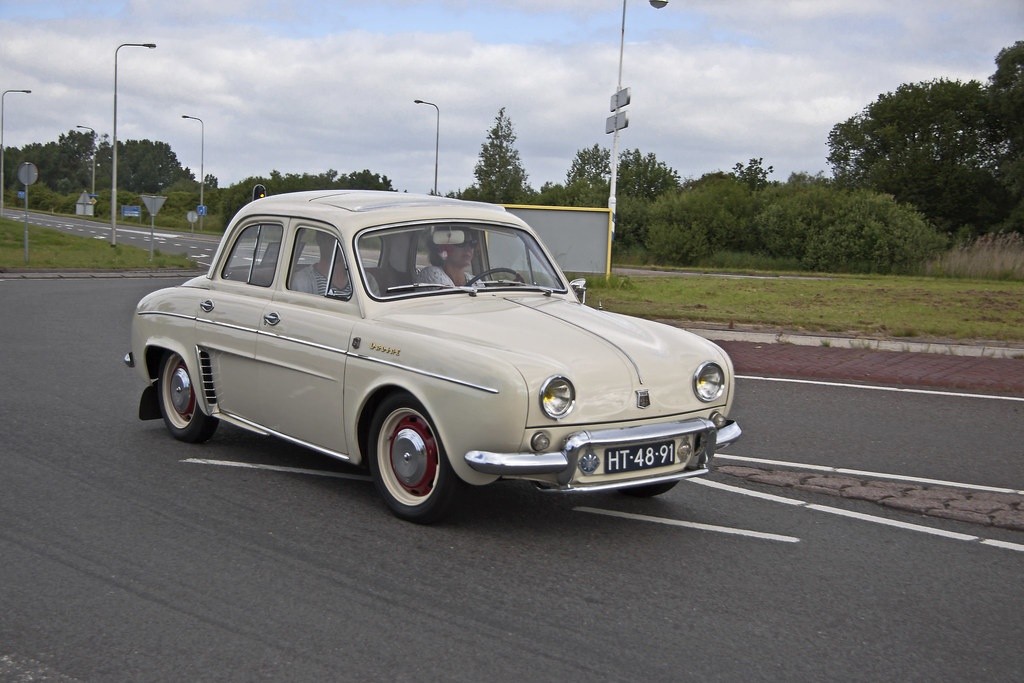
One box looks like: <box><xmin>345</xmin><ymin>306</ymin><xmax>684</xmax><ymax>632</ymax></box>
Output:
<box><xmin>456</xmin><ymin>240</ymin><xmax>478</xmax><ymax>249</ymax></box>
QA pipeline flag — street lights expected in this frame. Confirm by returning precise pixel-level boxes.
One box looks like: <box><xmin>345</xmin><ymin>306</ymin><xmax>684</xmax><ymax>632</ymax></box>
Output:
<box><xmin>1</xmin><ymin>89</ymin><xmax>32</xmax><ymax>215</ymax></box>
<box><xmin>182</xmin><ymin>114</ymin><xmax>204</xmax><ymax>229</ymax></box>
<box><xmin>77</xmin><ymin>125</ymin><xmax>95</xmax><ymax>195</ymax></box>
<box><xmin>109</xmin><ymin>41</ymin><xmax>156</xmax><ymax>245</ymax></box>
<box><xmin>414</xmin><ymin>97</ymin><xmax>440</xmax><ymax>194</ymax></box>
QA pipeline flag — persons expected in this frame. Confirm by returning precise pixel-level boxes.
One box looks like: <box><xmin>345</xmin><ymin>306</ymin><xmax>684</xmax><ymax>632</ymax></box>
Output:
<box><xmin>417</xmin><ymin>225</ymin><xmax>486</xmax><ymax>289</ymax></box>
<box><xmin>291</xmin><ymin>230</ymin><xmax>380</xmax><ymax>300</ymax></box>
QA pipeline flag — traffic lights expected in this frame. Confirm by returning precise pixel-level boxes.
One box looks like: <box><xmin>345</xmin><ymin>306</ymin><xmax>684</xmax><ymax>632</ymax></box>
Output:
<box><xmin>252</xmin><ymin>183</ymin><xmax>267</xmax><ymax>201</ymax></box>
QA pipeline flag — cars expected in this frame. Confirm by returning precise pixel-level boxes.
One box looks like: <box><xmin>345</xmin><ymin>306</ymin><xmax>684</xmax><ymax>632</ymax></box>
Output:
<box><xmin>124</xmin><ymin>189</ymin><xmax>742</xmax><ymax>522</ymax></box>
